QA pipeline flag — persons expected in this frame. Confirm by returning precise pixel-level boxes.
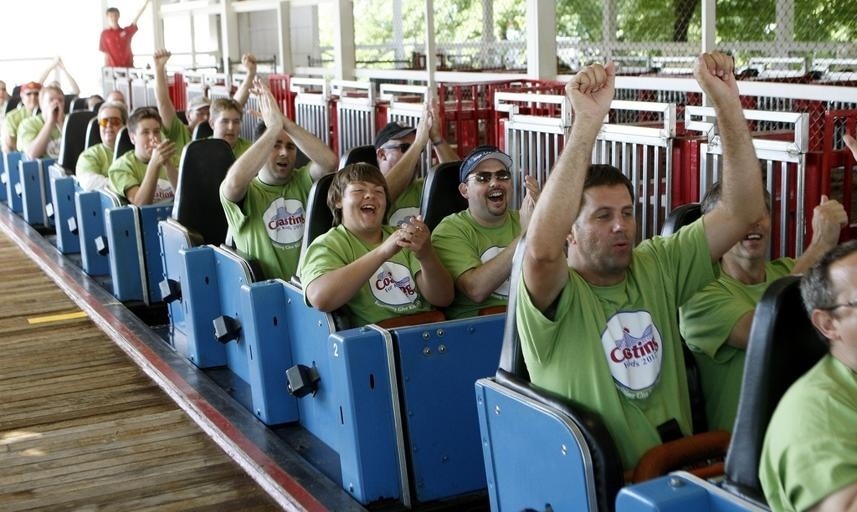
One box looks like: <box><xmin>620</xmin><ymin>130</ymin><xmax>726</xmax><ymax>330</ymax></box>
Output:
<box><xmin>298</xmin><ymin>162</ymin><xmax>456</xmax><ymax>326</ymax></box>
<box><xmin>372</xmin><ymin>98</ymin><xmax>462</xmax><ymax>227</ymax></box>
<box><xmin>100</xmin><ymin>0</ymin><xmax>152</xmax><ymax>69</ymax></box>
<box><xmin>16</xmin><ymin>86</ymin><xmax>71</xmax><ymax>159</ymax></box>
<box><xmin>75</xmin><ymin>101</ymin><xmax>128</xmax><ymax>192</ymax></box>
<box><xmin>0</xmin><ymin>81</ymin><xmax>9</xmax><ymax>120</ymax></box>
<box><xmin>206</xmin><ymin>98</ymin><xmax>253</xmax><ymax>158</ymax></box>
<box><xmin>153</xmin><ymin>49</ymin><xmax>259</xmax><ymax>151</ymax></box>
<box><xmin>38</xmin><ymin>58</ymin><xmax>80</xmax><ymax>115</ymax></box>
<box><xmin>677</xmin><ymin>176</ymin><xmax>848</xmax><ymax>433</ymax></box>
<box><xmin>758</xmin><ymin>238</ymin><xmax>857</xmax><ymax>512</ymax></box>
<box><xmin>2</xmin><ymin>81</ymin><xmax>45</xmax><ymax>153</ymax></box>
<box><xmin>109</xmin><ymin>107</ymin><xmax>180</xmax><ymax>206</ymax></box>
<box><xmin>431</xmin><ymin>143</ymin><xmax>542</xmax><ymax>319</ymax></box>
<box><xmin>106</xmin><ymin>91</ymin><xmax>126</xmax><ymax>106</ymax></box>
<box><xmin>514</xmin><ymin>52</ymin><xmax>768</xmax><ymax>484</ymax></box>
<box><xmin>218</xmin><ymin>79</ymin><xmax>339</xmax><ymax>279</ymax></box>
<box><xmin>85</xmin><ymin>95</ymin><xmax>104</xmax><ymax>110</ymax></box>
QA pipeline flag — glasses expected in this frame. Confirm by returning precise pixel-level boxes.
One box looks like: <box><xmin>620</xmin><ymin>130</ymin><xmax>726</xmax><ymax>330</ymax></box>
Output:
<box><xmin>383</xmin><ymin>142</ymin><xmax>424</xmax><ymax>154</ymax></box>
<box><xmin>98</xmin><ymin>117</ymin><xmax>124</xmax><ymax>126</ymax></box>
<box><xmin>821</xmin><ymin>299</ymin><xmax>857</xmax><ymax>311</ymax></box>
<box><xmin>464</xmin><ymin>169</ymin><xmax>511</xmax><ymax>183</ymax></box>
<box><xmin>26</xmin><ymin>92</ymin><xmax>38</xmax><ymax>95</ymax></box>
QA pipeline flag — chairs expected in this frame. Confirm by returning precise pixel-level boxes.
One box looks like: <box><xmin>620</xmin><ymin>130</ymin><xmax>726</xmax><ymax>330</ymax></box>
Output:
<box><xmin>112</xmin><ymin>127</ymin><xmax>134</xmax><ymax>161</ymax></box>
<box><xmin>191</xmin><ymin>121</ymin><xmax>214</xmax><ymax>140</ymax></box>
<box><xmin>172</xmin><ymin>137</ymin><xmax>238</xmax><ymax>246</ymax></box>
<box><xmin>724</xmin><ymin>272</ymin><xmax>830</xmax><ymax>510</ymax></box>
<box><xmin>339</xmin><ymin>144</ymin><xmax>378</xmax><ymax>171</ymax></box>
<box><xmin>660</xmin><ymin>203</ymin><xmax>701</xmax><ymax>238</ymax></box>
<box><xmin>84</xmin><ymin>117</ymin><xmax>102</xmax><ymax>150</ymax></box>
<box><xmin>57</xmin><ymin>109</ymin><xmax>97</xmax><ymax>171</ymax></box>
<box><xmin>296</xmin><ymin>169</ymin><xmax>335</xmax><ymax>280</ymax></box>
<box><xmin>420</xmin><ymin>160</ymin><xmax>469</xmax><ymax>237</ymax></box>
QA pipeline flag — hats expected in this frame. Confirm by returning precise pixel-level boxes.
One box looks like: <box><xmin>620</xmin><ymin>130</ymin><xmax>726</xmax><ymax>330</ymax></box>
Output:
<box><xmin>459</xmin><ymin>144</ymin><xmax>513</xmax><ymax>182</ymax></box>
<box><xmin>185</xmin><ymin>96</ymin><xmax>211</xmax><ymax>111</ymax></box>
<box><xmin>374</xmin><ymin>121</ymin><xmax>417</xmax><ymax>150</ymax></box>
<box><xmin>19</xmin><ymin>81</ymin><xmax>41</xmax><ymax>91</ymax></box>
